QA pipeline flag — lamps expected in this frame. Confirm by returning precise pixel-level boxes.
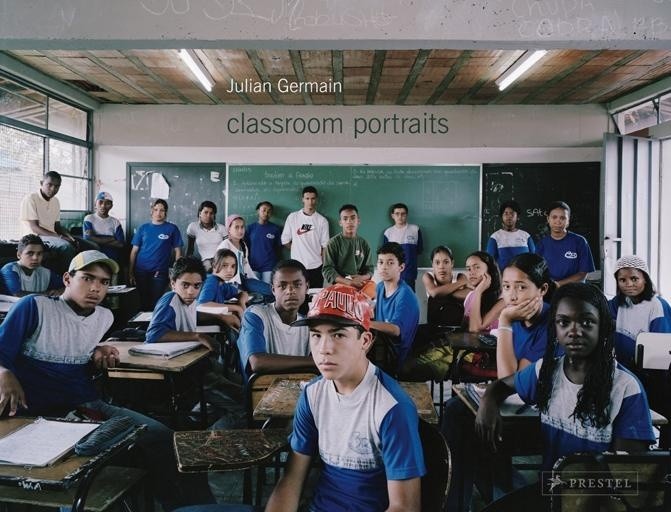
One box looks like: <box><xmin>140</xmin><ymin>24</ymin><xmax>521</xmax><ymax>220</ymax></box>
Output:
<box><xmin>177</xmin><ymin>47</ymin><xmax>216</xmax><ymax>92</ymax></box>
<box><xmin>492</xmin><ymin>48</ymin><xmax>547</xmax><ymax>92</ymax></box>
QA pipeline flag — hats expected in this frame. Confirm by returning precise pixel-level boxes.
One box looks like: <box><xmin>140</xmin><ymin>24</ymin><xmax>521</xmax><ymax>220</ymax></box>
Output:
<box><xmin>614</xmin><ymin>257</ymin><xmax>649</xmax><ymax>275</ymax></box>
<box><xmin>226</xmin><ymin>215</ymin><xmax>240</xmax><ymax>232</ymax></box>
<box><xmin>68</xmin><ymin>250</ymin><xmax>119</xmax><ymax>273</ymax></box>
<box><xmin>95</xmin><ymin>192</ymin><xmax>113</xmax><ymax>201</ymax></box>
<box><xmin>290</xmin><ymin>285</ymin><xmax>371</xmax><ymax>331</ymax></box>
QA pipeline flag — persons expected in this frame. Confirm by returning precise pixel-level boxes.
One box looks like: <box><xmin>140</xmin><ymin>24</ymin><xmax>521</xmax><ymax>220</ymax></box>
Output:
<box><xmin>280</xmin><ymin>183</ymin><xmax>331</xmax><ymax>288</ymax></box>
<box><xmin>82</xmin><ymin>190</ymin><xmax>124</xmax><ymax>282</ymax></box>
<box><xmin>18</xmin><ymin>170</ymin><xmax>101</xmax><ymax>271</ymax></box>
<box><xmin>535</xmin><ymin>199</ymin><xmax>597</xmax><ymax>292</ymax></box>
<box><xmin>382</xmin><ymin>202</ymin><xmax>423</xmax><ymax>295</ymax></box>
<box><xmin>321</xmin><ymin>204</ymin><xmax>375</xmax><ymax>289</ymax></box>
<box><xmin>1</xmin><ymin>234</ymin><xmax>671</xmax><ymax>510</ymax></box>
<box><xmin>484</xmin><ymin>200</ymin><xmax>537</xmax><ymax>274</ymax></box>
<box><xmin>217</xmin><ymin>213</ymin><xmax>273</xmax><ymax>299</ymax></box>
<box><xmin>188</xmin><ymin>200</ymin><xmax>229</xmax><ymax>272</ymax></box>
<box><xmin>128</xmin><ymin>198</ymin><xmax>184</xmax><ymax>312</ymax></box>
<box><xmin>244</xmin><ymin>200</ymin><xmax>282</xmax><ymax>283</ymax></box>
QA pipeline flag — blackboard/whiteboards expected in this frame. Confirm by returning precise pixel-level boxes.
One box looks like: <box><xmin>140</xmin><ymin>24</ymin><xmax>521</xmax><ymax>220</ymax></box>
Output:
<box><xmin>126</xmin><ymin>161</ymin><xmax>225</xmax><ymax>260</ymax></box>
<box><xmin>481</xmin><ymin>161</ymin><xmax>601</xmax><ymax>271</ymax></box>
<box><xmin>225</xmin><ymin>163</ymin><xmax>483</xmax><ymax>272</ymax></box>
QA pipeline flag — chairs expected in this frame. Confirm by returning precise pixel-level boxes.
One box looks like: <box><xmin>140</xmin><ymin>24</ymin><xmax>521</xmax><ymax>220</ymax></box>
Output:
<box><xmin>0</xmin><ymin>277</ymin><xmax>671</xmax><ymax>512</ymax></box>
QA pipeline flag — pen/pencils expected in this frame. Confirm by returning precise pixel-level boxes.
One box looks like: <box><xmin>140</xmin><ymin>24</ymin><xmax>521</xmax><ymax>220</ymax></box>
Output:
<box><xmin>516</xmin><ymin>403</ymin><xmax>530</xmax><ymax>414</ymax></box>
